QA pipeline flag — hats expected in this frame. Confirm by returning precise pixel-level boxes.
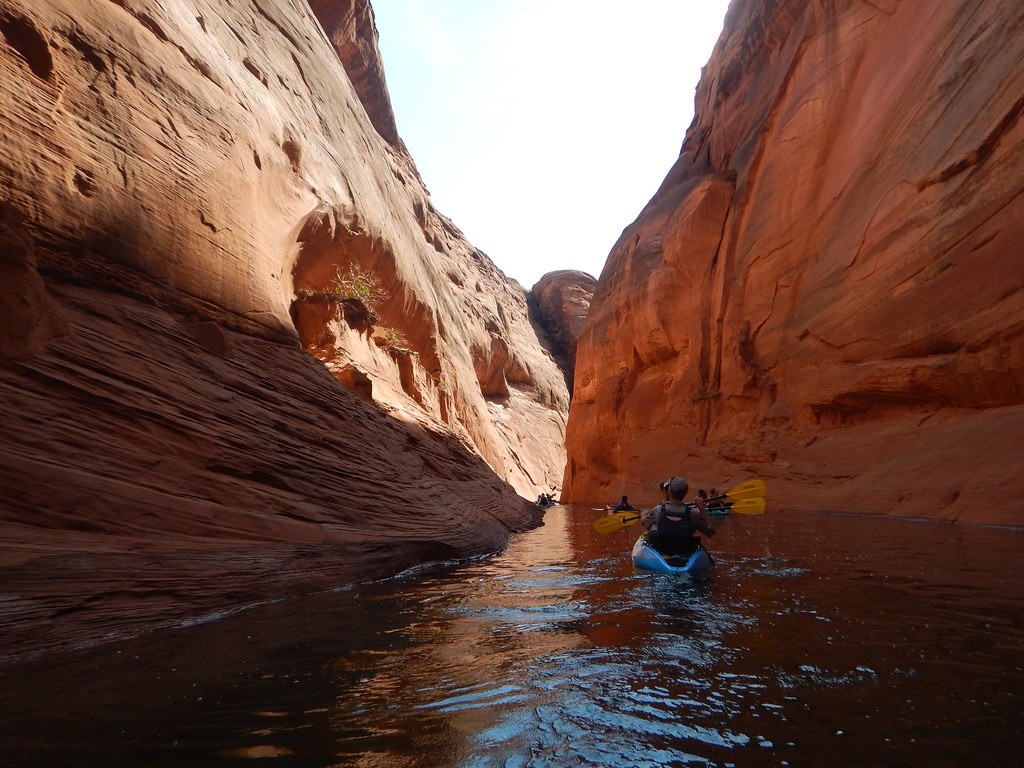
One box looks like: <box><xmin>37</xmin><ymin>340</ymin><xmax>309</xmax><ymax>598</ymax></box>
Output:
<box><xmin>670</xmin><ymin>477</ymin><xmax>688</xmax><ymax>494</ymax></box>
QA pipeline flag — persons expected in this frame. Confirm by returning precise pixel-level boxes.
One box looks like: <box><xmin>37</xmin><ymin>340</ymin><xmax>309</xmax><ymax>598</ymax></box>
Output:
<box><xmin>538</xmin><ymin>493</ymin><xmax>552</xmax><ymax>506</ymax></box>
<box><xmin>611</xmin><ymin>496</ymin><xmax>633</xmax><ymax>513</ymax></box>
<box><xmin>697</xmin><ymin>488</ymin><xmax>725</xmax><ymax>511</ymax></box>
<box><xmin>640</xmin><ymin>477</ymin><xmax>714</xmax><ymax>553</ymax></box>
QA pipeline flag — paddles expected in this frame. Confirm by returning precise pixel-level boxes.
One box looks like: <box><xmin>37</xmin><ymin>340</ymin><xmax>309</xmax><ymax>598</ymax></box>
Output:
<box><xmin>550</xmin><ymin>493</ymin><xmax>556</xmax><ymax>497</ymax></box>
<box><xmin>591</xmin><ymin>508</ymin><xmax>614</xmax><ymax>511</ymax></box>
<box><xmin>593</xmin><ymin>480</ymin><xmax>767</xmax><ymax>535</ymax></box>
<box><xmin>613</xmin><ymin>498</ymin><xmax>766</xmax><ymax>527</ymax></box>
<box><xmin>549</xmin><ymin>486</ymin><xmax>556</xmax><ymax>494</ymax></box>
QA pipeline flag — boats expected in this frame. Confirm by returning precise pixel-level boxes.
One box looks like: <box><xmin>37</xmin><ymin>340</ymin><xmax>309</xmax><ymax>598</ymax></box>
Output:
<box><xmin>606</xmin><ymin>504</ymin><xmax>640</xmax><ymax>516</ymax></box>
<box><xmin>631</xmin><ymin>530</ymin><xmax>714</xmax><ymax>576</ymax></box>
<box><xmin>710</xmin><ymin>506</ymin><xmax>730</xmax><ymax>515</ymax></box>
